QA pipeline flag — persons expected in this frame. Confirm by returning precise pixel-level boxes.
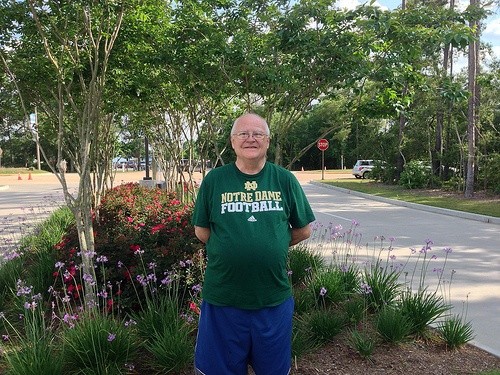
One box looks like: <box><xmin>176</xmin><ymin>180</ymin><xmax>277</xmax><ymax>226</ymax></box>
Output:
<box><xmin>191</xmin><ymin>113</ymin><xmax>315</xmax><ymax>375</ymax></box>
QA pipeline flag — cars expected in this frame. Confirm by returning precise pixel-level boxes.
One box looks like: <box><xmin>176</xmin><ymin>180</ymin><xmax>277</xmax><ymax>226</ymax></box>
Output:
<box><xmin>412</xmin><ymin>159</ymin><xmax>458</xmax><ymax>181</ymax></box>
<box><xmin>111</xmin><ymin>157</ymin><xmax>152</xmax><ymax>171</ymax></box>
<box><xmin>176</xmin><ymin>158</ymin><xmax>204</xmax><ymax>173</ymax></box>
<box><xmin>352</xmin><ymin>159</ymin><xmax>398</xmax><ymax>180</ymax></box>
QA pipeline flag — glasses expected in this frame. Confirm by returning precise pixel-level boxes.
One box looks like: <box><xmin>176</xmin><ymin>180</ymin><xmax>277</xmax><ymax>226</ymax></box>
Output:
<box><xmin>233</xmin><ymin>131</ymin><xmax>268</xmax><ymax>141</ymax></box>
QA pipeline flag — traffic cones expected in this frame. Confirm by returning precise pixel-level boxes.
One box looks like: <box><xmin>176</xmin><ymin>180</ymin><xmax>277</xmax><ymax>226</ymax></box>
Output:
<box><xmin>17</xmin><ymin>171</ymin><xmax>22</xmax><ymax>180</ymax></box>
<box><xmin>28</xmin><ymin>172</ymin><xmax>33</xmax><ymax>180</ymax></box>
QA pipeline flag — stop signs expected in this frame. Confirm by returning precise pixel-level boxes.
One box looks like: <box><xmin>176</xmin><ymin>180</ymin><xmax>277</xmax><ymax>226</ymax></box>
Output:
<box><xmin>316</xmin><ymin>139</ymin><xmax>330</xmax><ymax>151</ymax></box>
